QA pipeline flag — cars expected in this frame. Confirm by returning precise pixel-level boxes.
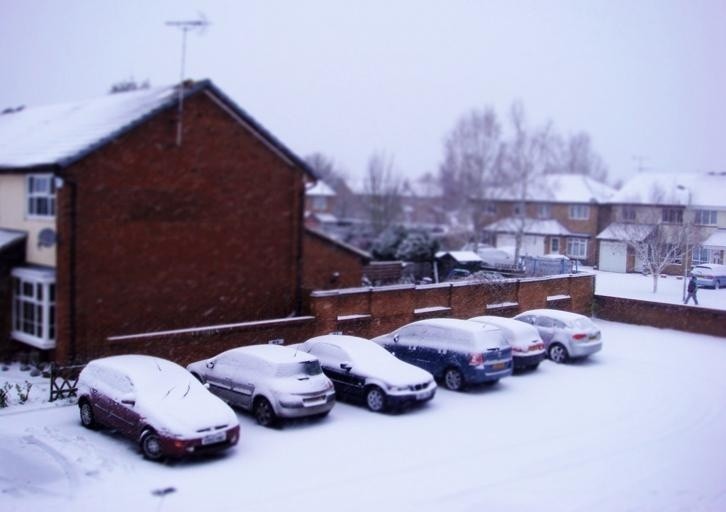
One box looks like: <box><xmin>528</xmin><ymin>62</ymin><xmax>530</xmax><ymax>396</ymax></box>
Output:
<box><xmin>513</xmin><ymin>307</ymin><xmax>605</xmax><ymax>365</ymax></box>
<box><xmin>288</xmin><ymin>331</ymin><xmax>441</xmax><ymax>414</ymax></box>
<box><xmin>73</xmin><ymin>354</ymin><xmax>242</xmax><ymax>464</ymax></box>
<box><xmin>467</xmin><ymin>314</ymin><xmax>548</xmax><ymax>376</ymax></box>
<box><xmin>184</xmin><ymin>343</ymin><xmax>336</xmax><ymax>429</ymax></box>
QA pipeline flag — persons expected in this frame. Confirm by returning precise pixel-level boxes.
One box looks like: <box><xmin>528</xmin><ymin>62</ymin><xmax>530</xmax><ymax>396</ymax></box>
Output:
<box><xmin>683</xmin><ymin>276</ymin><xmax>699</xmax><ymax>305</ymax></box>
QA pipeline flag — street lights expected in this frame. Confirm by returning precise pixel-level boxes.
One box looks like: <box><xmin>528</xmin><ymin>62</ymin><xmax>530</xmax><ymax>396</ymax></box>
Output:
<box><xmin>676</xmin><ymin>182</ymin><xmax>695</xmax><ymax>278</ymax></box>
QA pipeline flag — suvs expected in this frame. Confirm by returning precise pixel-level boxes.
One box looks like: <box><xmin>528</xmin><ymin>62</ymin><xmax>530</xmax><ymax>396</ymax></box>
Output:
<box><xmin>690</xmin><ymin>262</ymin><xmax>726</xmax><ymax>291</ymax></box>
<box><xmin>369</xmin><ymin>317</ymin><xmax>516</xmax><ymax>393</ymax></box>
